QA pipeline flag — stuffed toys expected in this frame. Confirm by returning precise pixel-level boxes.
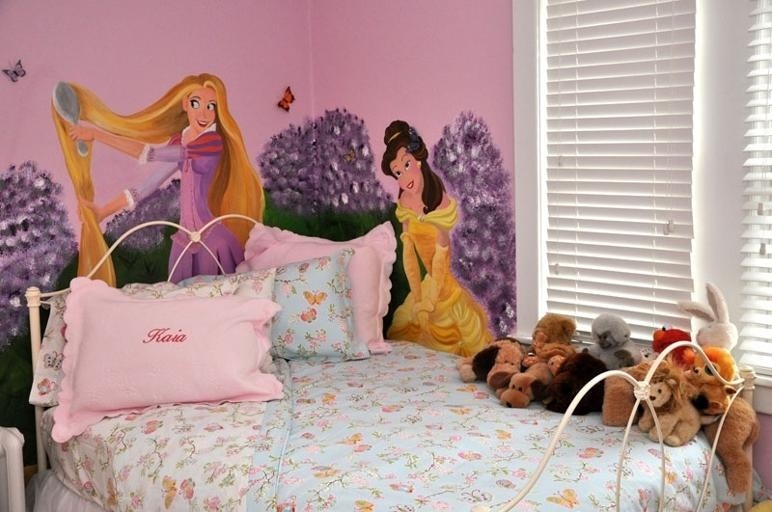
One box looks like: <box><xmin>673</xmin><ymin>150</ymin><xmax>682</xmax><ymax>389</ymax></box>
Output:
<box><xmin>676</xmin><ymin>281</ymin><xmax>742</xmax><ymax>392</ymax></box>
<box><xmin>459</xmin><ymin>312</ymin><xmax>745</xmax><ymax>448</ymax></box>
<box><xmin>702</xmin><ymin>395</ymin><xmax>759</xmax><ymax>493</ymax></box>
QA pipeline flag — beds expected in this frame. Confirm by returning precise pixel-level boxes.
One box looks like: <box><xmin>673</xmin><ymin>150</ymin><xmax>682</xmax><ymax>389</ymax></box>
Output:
<box><xmin>25</xmin><ymin>214</ymin><xmax>756</xmax><ymax>511</ymax></box>
